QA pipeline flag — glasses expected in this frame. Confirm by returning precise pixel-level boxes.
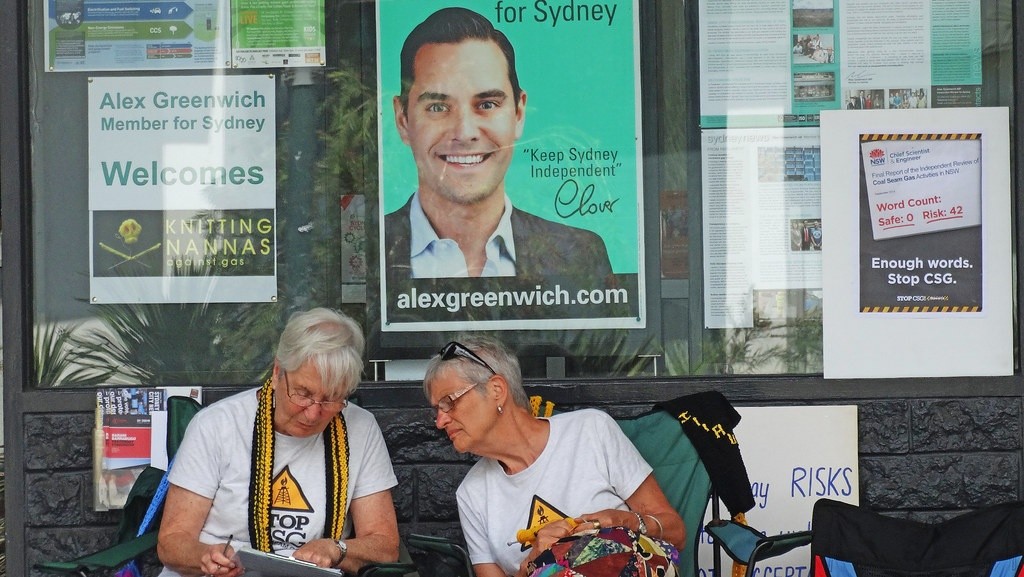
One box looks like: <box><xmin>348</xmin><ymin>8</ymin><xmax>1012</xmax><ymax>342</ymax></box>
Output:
<box><xmin>437</xmin><ymin>341</ymin><xmax>498</xmax><ymax>376</ymax></box>
<box><xmin>283</xmin><ymin>370</ymin><xmax>350</xmax><ymax>412</ymax></box>
<box><xmin>426</xmin><ymin>381</ymin><xmax>487</xmax><ymax>421</ymax></box>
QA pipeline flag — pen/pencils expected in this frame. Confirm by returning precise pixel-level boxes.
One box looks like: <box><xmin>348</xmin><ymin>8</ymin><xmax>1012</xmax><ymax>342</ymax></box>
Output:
<box><xmin>213</xmin><ymin>534</ymin><xmax>234</xmax><ymax>572</ymax></box>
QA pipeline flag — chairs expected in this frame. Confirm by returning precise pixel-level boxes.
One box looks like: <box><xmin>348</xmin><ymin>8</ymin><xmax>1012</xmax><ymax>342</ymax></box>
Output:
<box><xmin>33</xmin><ymin>396</ymin><xmax>419</xmax><ymax>577</ymax></box>
<box><xmin>809</xmin><ymin>498</ymin><xmax>1024</xmax><ymax>577</ymax></box>
<box><xmin>406</xmin><ymin>390</ymin><xmax>812</xmax><ymax>577</ymax></box>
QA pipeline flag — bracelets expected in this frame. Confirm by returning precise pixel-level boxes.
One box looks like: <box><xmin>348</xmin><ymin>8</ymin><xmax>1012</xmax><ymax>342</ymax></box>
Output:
<box><xmin>645</xmin><ymin>514</ymin><xmax>664</xmax><ymax>542</ymax></box>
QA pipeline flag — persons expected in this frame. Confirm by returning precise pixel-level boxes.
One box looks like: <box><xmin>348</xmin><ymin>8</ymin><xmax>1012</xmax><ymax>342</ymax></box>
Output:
<box><xmin>156</xmin><ymin>307</ymin><xmax>401</xmax><ymax>576</ymax></box>
<box><xmin>790</xmin><ymin>219</ymin><xmax>823</xmax><ymax>251</ymax></box>
<box><xmin>793</xmin><ymin>34</ymin><xmax>835</xmax><ymax>65</ymax></box>
<box><xmin>422</xmin><ymin>332</ymin><xmax>687</xmax><ymax>577</ymax></box>
<box><xmin>846</xmin><ymin>91</ymin><xmax>883</xmax><ymax>109</ymax></box>
<box><xmin>889</xmin><ymin>89</ymin><xmax>926</xmax><ymax>109</ymax></box>
<box><xmin>385</xmin><ymin>6</ymin><xmax>638</xmax><ymax>298</ymax></box>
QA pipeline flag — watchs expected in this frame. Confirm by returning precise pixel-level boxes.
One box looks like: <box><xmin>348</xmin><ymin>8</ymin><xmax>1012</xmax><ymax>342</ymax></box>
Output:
<box><xmin>634</xmin><ymin>513</ymin><xmax>648</xmax><ymax>535</ymax></box>
<box><xmin>329</xmin><ymin>540</ymin><xmax>347</xmax><ymax>568</ymax></box>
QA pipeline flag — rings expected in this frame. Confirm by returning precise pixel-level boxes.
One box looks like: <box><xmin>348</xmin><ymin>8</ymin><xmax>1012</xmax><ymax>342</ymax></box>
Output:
<box><xmin>592</xmin><ymin>519</ymin><xmax>601</xmax><ymax>529</ymax></box>
